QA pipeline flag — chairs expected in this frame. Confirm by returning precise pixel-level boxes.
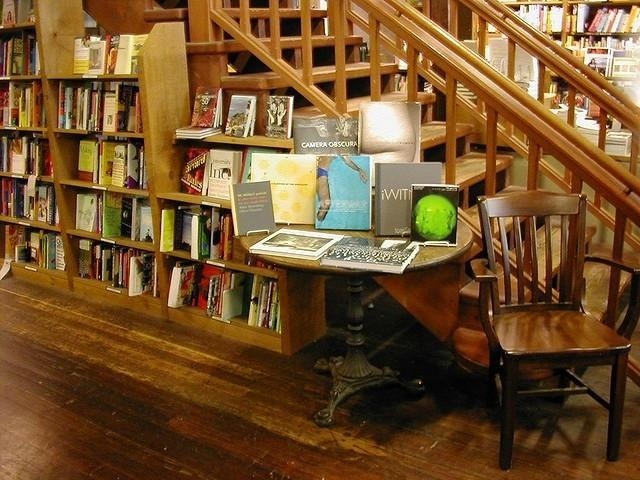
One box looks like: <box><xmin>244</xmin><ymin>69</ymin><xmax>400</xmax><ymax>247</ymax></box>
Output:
<box><xmin>476</xmin><ymin>191</ymin><xmax>632</xmax><ymax>470</ymax></box>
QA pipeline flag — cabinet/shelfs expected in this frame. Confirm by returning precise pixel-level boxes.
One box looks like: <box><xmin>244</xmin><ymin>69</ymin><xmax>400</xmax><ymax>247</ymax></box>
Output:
<box><xmin>565</xmin><ymin>0</ymin><xmax>640</xmax><ymax>80</ymax></box>
<box><xmin>0</xmin><ymin>0</ymin><xmax>86</xmax><ymax>297</ymax></box>
<box><xmin>157</xmin><ymin>134</ymin><xmax>326</xmax><ymax>357</ymax></box>
<box><xmin>47</xmin><ymin>21</ymin><xmax>192</xmax><ymax>322</ymax></box>
<box><xmin>489</xmin><ymin>0</ymin><xmax>565</xmax><ymax>77</ymax></box>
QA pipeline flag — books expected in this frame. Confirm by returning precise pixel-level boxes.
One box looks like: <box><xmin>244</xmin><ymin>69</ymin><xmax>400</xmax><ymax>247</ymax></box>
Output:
<box><xmin>293</xmin><ymin>118</ymin><xmax>359</xmax><ymax>156</ymax></box>
<box><xmin>411</xmin><ymin>183</ymin><xmax>459</xmax><ymax>247</ymax></box>
<box><xmin>63</xmin><ymin>34</ymin><xmax>159</xmax><ymax>297</ymax></box>
<box><xmin>252</xmin><ymin>153</ymin><xmax>372</xmax><ymax>230</ymax></box>
<box><xmin>484</xmin><ymin>1</ymin><xmax>640</xmax><ymax>157</ymax></box>
<box><xmin>374</xmin><ymin>163</ymin><xmax>442</xmax><ymax>236</ymax></box>
<box><xmin>264</xmin><ymin>94</ymin><xmax>294</xmax><ymax>139</ymax></box>
<box><xmin>358</xmin><ymin>101</ymin><xmax>421</xmax><ymax>186</ymax></box>
<box><xmin>319</xmin><ymin>235</ymin><xmax>419</xmax><ymax>274</ymax></box>
<box><xmin>248</xmin><ymin>229</ymin><xmax>345</xmax><ymax>261</ymax></box>
<box><xmin>159</xmin><ymin>85</ymin><xmax>281</xmax><ymax>333</ymax></box>
<box><xmin>2</xmin><ymin>0</ymin><xmax>66</xmax><ymax>271</ymax></box>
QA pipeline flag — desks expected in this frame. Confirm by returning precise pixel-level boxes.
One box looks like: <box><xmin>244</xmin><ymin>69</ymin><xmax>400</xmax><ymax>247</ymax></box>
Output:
<box><xmin>240</xmin><ymin>217</ymin><xmax>475</xmax><ymax>425</ymax></box>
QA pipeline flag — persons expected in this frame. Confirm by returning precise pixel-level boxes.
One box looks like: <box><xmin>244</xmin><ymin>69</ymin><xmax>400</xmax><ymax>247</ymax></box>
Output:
<box><xmin>85</xmin><ymin>34</ymin><xmax>91</xmax><ymax>47</ymax></box>
<box><xmin>221</xmin><ymin>168</ymin><xmax>231</xmax><ymax>180</ymax></box>
<box><xmin>316</xmin><ymin>156</ymin><xmax>369</xmax><ymax>227</ymax></box>
<box><xmin>38</xmin><ymin>198</ymin><xmax>47</xmax><ymax>217</ymax></box>
<box><xmin>81</xmin><ymin>37</ymin><xmax>86</xmax><ymax>46</ymax></box>
<box><xmin>249</xmin><ymin>297</ymin><xmax>258</xmax><ymax>326</ymax></box>
<box><xmin>107</xmin><ymin>115</ymin><xmax>113</xmax><ymax>124</ymax></box>
<box><xmin>180</xmin><ymin>272</ymin><xmax>190</xmax><ymax>298</ymax></box>
